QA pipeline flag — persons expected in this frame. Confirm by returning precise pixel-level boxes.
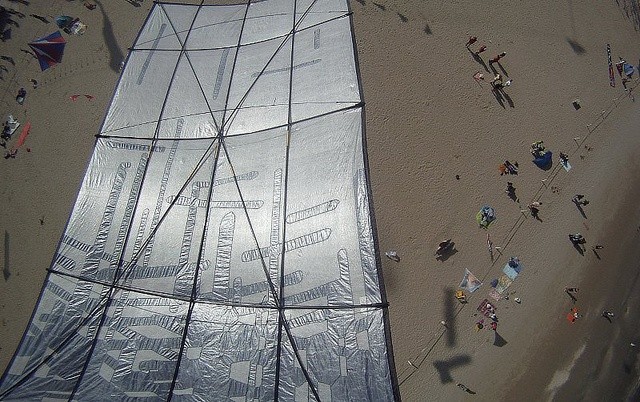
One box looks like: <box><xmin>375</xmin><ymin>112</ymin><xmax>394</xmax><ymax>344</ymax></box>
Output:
<box><xmin>491</xmin><ymin>74</ymin><xmax>501</xmax><ymax>85</ymax></box>
<box><xmin>489</xmin><ymin>49</ymin><xmax>507</xmax><ymax>66</ymax></box>
<box><xmin>464</xmin><ymin>34</ymin><xmax>478</xmax><ymax>49</ymax></box>
<box><xmin>527</xmin><ymin>202</ymin><xmax>543</xmax><ymax>209</ymax></box>
<box><xmin>504</xmin><ymin>80</ymin><xmax>513</xmax><ymax>88</ymax></box>
<box><xmin>578</xmin><ymin>201</ymin><xmax>590</xmax><ymax>207</ymax></box>
<box><xmin>474</xmin><ymin>43</ymin><xmax>486</xmax><ymax>55</ymax></box>
<box><xmin>568</xmin><ymin>232</ymin><xmax>582</xmax><ymax>239</ymax></box>
<box><xmin>573</xmin><ymin>194</ymin><xmax>585</xmax><ymax>200</ymax></box>
